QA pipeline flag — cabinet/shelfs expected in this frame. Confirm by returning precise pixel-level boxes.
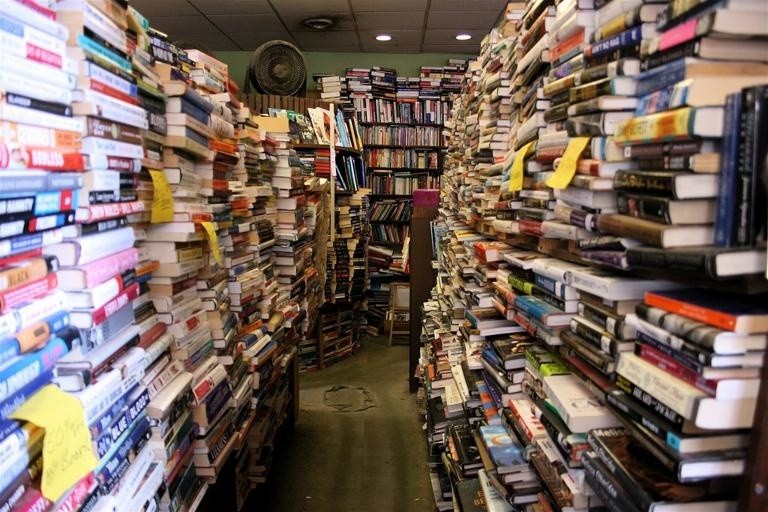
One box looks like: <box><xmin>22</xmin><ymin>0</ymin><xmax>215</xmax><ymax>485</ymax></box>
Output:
<box><xmin>207</xmin><ymin>344</ymin><xmax>301</xmax><ymax>512</ymax></box>
<box><xmin>286</xmin><ymin>89</ymin><xmax>458</xmax><ymax>397</ymax></box>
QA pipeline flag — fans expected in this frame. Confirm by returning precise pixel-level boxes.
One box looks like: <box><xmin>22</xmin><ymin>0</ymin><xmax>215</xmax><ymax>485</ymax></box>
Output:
<box><xmin>242</xmin><ymin>40</ymin><xmax>308</xmax><ymax>98</ymax></box>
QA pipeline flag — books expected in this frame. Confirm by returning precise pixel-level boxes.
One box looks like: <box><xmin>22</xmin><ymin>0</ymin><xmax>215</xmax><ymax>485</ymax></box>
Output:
<box><xmin>293</xmin><ymin>60</ymin><xmax>412</xmax><ymax>374</ymax></box>
<box><xmin>0</xmin><ymin>0</ymin><xmax>297</xmax><ymax>512</ymax></box>
<box><xmin>412</xmin><ymin>1</ymin><xmax>768</xmax><ymax>511</ymax></box>
